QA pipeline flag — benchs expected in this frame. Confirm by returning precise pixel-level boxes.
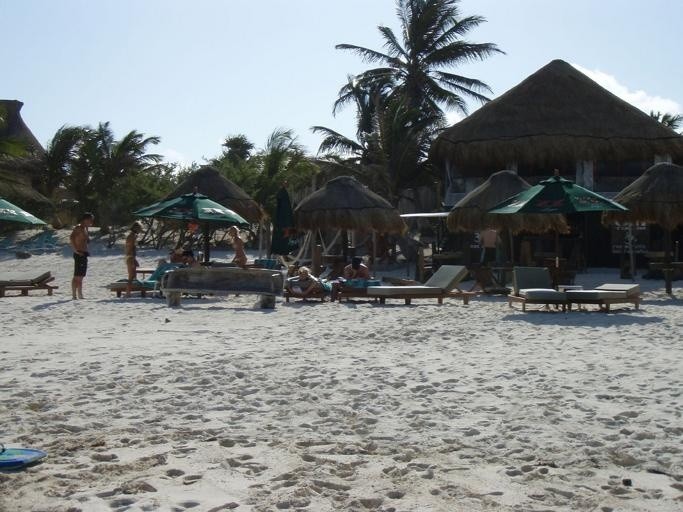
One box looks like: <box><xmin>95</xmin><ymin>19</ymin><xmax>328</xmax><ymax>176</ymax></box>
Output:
<box><xmin>161</xmin><ymin>267</ymin><xmax>286</xmax><ymax>310</ymax></box>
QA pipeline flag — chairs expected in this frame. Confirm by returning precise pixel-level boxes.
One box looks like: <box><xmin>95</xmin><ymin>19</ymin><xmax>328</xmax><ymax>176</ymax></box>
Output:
<box><xmin>105</xmin><ymin>262</ymin><xmax>181</xmax><ymax>298</ymax></box>
<box><xmin>507</xmin><ymin>265</ymin><xmax>643</xmax><ymax>315</ymax></box>
<box><xmin>0</xmin><ymin>271</ymin><xmax>58</xmax><ymax>297</ymax></box>
<box><xmin>364</xmin><ymin>264</ymin><xmax>475</xmax><ymax>307</ymax></box>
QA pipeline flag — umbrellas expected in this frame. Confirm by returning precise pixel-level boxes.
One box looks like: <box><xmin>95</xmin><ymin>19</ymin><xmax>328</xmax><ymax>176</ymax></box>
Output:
<box><xmin>600</xmin><ymin>161</ymin><xmax>682</xmax><ymax>293</ymax></box>
<box><xmin>427</xmin><ymin>59</ymin><xmax>683</xmax><ymax>165</ymax></box>
<box><xmin>291</xmin><ymin>175</ymin><xmax>408</xmax><ymax>264</ymax></box>
<box><xmin>446</xmin><ymin>169</ymin><xmax>572</xmax><ymax>288</ymax></box>
<box><xmin>129</xmin><ymin>184</ymin><xmax>250</xmax><ymax>228</ymax></box>
<box><xmin>482</xmin><ymin>168</ymin><xmax>631</xmax><ymax>308</ymax></box>
<box><xmin>156</xmin><ymin>164</ymin><xmax>270</xmax><ymax>261</ymax></box>
<box><xmin>0</xmin><ymin>195</ymin><xmax>46</xmax><ymax>225</ymax></box>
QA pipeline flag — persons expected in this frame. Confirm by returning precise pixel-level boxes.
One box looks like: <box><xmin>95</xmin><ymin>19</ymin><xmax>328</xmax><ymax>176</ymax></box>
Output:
<box><xmin>123</xmin><ymin>221</ymin><xmax>144</xmax><ymax>301</ymax></box>
<box><xmin>226</xmin><ymin>225</ymin><xmax>247</xmax><ymax>268</ymax></box>
<box><xmin>342</xmin><ymin>257</ymin><xmax>369</xmax><ymax>279</ymax></box>
<box><xmin>284</xmin><ymin>266</ymin><xmax>330</xmax><ymax>297</ymax></box>
<box><xmin>193</xmin><ymin>245</ymin><xmax>203</xmax><ymax>261</ymax></box>
<box><xmin>478</xmin><ymin>226</ymin><xmax>501</xmax><ymax>264</ymax></box>
<box><xmin>68</xmin><ymin>211</ymin><xmax>94</xmax><ymax>300</ymax></box>
